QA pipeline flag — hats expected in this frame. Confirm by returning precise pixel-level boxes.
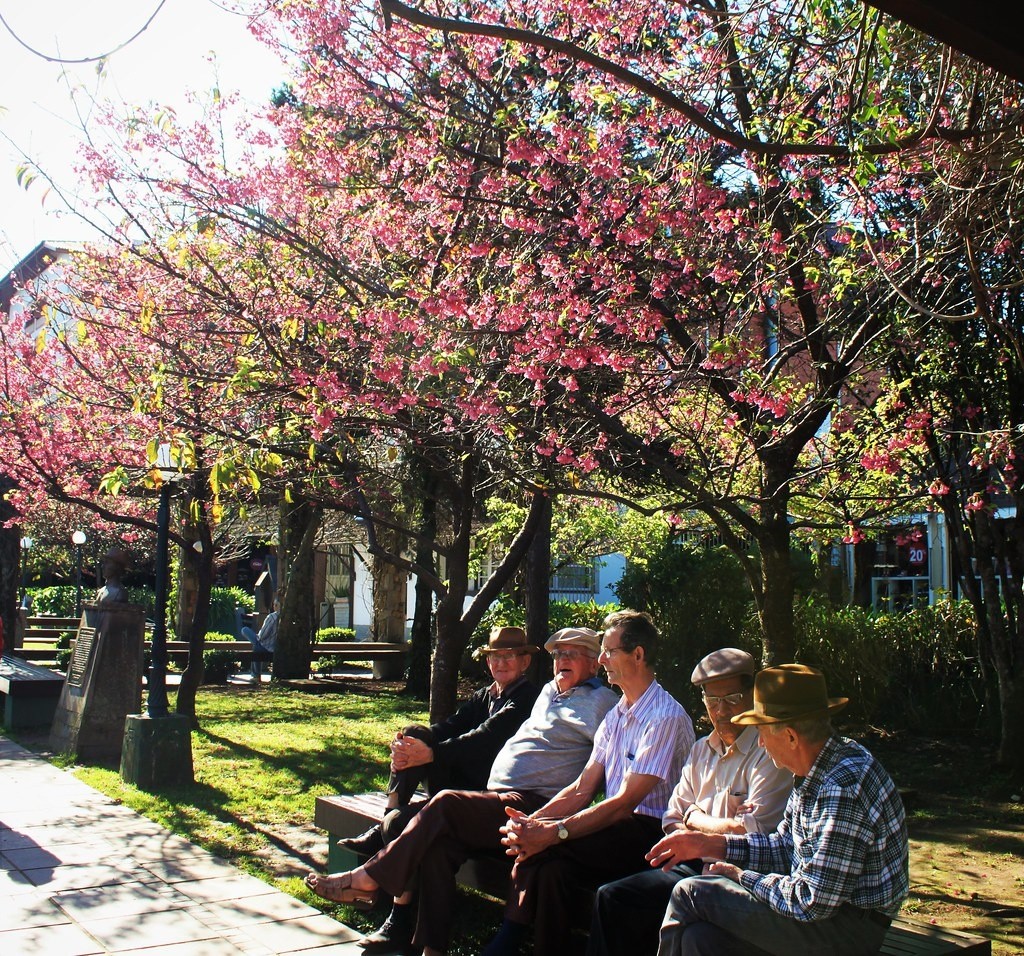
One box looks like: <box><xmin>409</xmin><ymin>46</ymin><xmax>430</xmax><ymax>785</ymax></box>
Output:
<box><xmin>690</xmin><ymin>648</ymin><xmax>755</xmax><ymax>686</ymax></box>
<box><xmin>478</xmin><ymin>626</ymin><xmax>540</xmax><ymax>654</ymax></box>
<box><xmin>544</xmin><ymin>628</ymin><xmax>601</xmax><ymax>654</ymax></box>
<box><xmin>730</xmin><ymin>664</ymin><xmax>849</xmax><ymax>725</ymax></box>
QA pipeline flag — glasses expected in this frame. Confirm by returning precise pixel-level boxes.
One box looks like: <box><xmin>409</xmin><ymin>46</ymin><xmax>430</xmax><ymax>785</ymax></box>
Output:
<box><xmin>702</xmin><ymin>690</ymin><xmax>754</xmax><ymax>708</ymax></box>
<box><xmin>488</xmin><ymin>652</ymin><xmax>528</xmax><ymax>661</ymax></box>
<box><xmin>600</xmin><ymin>645</ymin><xmax>638</xmax><ymax>659</ymax></box>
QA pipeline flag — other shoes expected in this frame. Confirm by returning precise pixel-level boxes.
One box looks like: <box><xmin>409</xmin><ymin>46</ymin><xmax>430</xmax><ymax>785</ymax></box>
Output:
<box><xmin>357</xmin><ymin>918</ymin><xmax>411</xmax><ymax>948</ymax></box>
<box><xmin>337</xmin><ymin>825</ymin><xmax>388</xmax><ymax>857</ymax></box>
<box><xmin>304</xmin><ymin>872</ymin><xmax>379</xmax><ymax>911</ymax></box>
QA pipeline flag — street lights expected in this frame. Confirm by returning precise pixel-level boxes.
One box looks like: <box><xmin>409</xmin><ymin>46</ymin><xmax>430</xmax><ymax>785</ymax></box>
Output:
<box><xmin>20</xmin><ymin>536</ymin><xmax>33</xmax><ymax>606</ymax></box>
<box><xmin>72</xmin><ymin>531</ymin><xmax>88</xmax><ymax>618</ymax></box>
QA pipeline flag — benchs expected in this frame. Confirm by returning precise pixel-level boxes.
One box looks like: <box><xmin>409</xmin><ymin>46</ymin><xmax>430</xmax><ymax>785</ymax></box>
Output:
<box><xmin>16</xmin><ymin>618</ymin><xmax>82</xmax><ymax>659</ymax></box>
<box><xmin>167</xmin><ymin>650</ymin><xmax>410</xmax><ymax>684</ymax></box>
<box><xmin>314</xmin><ymin>790</ymin><xmax>991</xmax><ymax>956</ymax></box>
<box><xmin>0</xmin><ymin>651</ymin><xmax>66</xmax><ymax>732</ymax></box>
<box><xmin>144</xmin><ymin>640</ymin><xmax>410</xmax><ymax>665</ymax></box>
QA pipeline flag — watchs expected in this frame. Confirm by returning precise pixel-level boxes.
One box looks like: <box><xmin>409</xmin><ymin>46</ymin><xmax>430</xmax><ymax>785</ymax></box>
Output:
<box><xmin>557</xmin><ymin>822</ymin><xmax>569</xmax><ymax>840</ymax></box>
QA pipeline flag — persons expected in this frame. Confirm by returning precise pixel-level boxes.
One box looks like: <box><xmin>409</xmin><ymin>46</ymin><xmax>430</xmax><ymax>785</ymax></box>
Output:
<box><xmin>304</xmin><ymin>612</ymin><xmax>909</xmax><ymax>956</ymax></box>
<box><xmin>96</xmin><ymin>547</ymin><xmax>129</xmax><ymax>603</ymax></box>
<box><xmin>241</xmin><ymin>598</ymin><xmax>278</xmax><ymax>683</ymax></box>
